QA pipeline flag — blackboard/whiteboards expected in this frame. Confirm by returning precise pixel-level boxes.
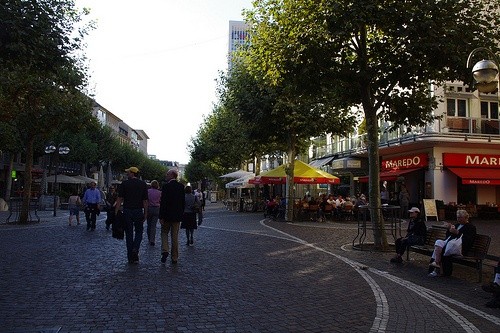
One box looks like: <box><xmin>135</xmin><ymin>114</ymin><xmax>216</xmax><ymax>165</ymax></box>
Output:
<box><xmin>423</xmin><ymin>198</ymin><xmax>437</xmax><ymax>216</ymax></box>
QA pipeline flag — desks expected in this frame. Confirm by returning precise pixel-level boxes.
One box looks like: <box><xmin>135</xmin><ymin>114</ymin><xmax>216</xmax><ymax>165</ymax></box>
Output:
<box><xmin>351</xmin><ymin>203</ymin><xmax>401</xmax><ymax>250</ymax></box>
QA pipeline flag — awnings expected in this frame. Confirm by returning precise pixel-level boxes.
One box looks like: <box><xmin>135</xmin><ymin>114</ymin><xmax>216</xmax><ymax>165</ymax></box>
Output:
<box><xmin>448</xmin><ymin>168</ymin><xmax>500</xmax><ymax>186</ymax></box>
<box><xmin>359</xmin><ymin>168</ymin><xmax>423</xmax><ymax>183</ymax></box>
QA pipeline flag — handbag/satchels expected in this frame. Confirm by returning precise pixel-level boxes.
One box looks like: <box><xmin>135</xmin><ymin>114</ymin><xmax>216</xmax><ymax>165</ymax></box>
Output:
<box><xmin>111</xmin><ymin>211</ymin><xmax>125</xmax><ymax>240</ymax></box>
<box><xmin>444</xmin><ymin>233</ymin><xmax>463</xmax><ymax>256</ymax></box>
<box><xmin>97</xmin><ymin>208</ymin><xmax>100</xmax><ymax>216</ymax></box>
<box><xmin>192</xmin><ymin>195</ymin><xmax>201</xmax><ymax>213</ymax></box>
<box><xmin>76</xmin><ymin>196</ymin><xmax>81</xmax><ymax>207</ymax></box>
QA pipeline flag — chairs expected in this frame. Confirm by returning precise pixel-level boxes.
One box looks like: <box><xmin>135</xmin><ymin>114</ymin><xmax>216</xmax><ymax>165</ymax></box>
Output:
<box><xmin>297</xmin><ymin>205</ymin><xmax>352</xmax><ymax>219</ymax></box>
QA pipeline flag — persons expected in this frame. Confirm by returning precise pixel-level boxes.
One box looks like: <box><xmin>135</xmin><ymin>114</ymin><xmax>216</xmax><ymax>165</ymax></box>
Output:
<box><xmin>147</xmin><ymin>180</ymin><xmax>161</xmax><ymax>247</ymax></box>
<box><xmin>264</xmin><ymin>193</ymin><xmax>367</xmax><ymax>222</ymax></box>
<box><xmin>158</xmin><ymin>169</ymin><xmax>186</xmax><ymax>265</ymax></box>
<box><xmin>390</xmin><ymin>207</ymin><xmax>428</xmax><ymax>263</ymax></box>
<box><xmin>191</xmin><ymin>187</ymin><xmax>206</xmax><ymax>211</ymax></box>
<box><xmin>398</xmin><ymin>188</ymin><xmax>410</xmax><ymax>219</ymax></box>
<box><xmin>427</xmin><ymin>210</ymin><xmax>476</xmax><ymax>281</ymax></box>
<box><xmin>481</xmin><ymin>261</ymin><xmax>500</xmax><ymax>308</ymax></box>
<box><xmin>180</xmin><ymin>186</ymin><xmax>202</xmax><ymax>246</ymax></box>
<box><xmin>68</xmin><ymin>190</ymin><xmax>82</xmax><ymax>226</ymax></box>
<box><xmin>82</xmin><ymin>181</ymin><xmax>118</xmax><ymax>232</ymax></box>
<box><xmin>114</xmin><ymin>167</ymin><xmax>149</xmax><ymax>264</ymax></box>
<box><xmin>380</xmin><ymin>186</ymin><xmax>390</xmax><ymax>204</ymax></box>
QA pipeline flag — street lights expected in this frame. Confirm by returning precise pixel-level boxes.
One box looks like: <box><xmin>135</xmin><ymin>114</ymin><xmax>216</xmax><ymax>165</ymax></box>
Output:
<box><xmin>45</xmin><ymin>141</ymin><xmax>71</xmax><ymax>217</ymax></box>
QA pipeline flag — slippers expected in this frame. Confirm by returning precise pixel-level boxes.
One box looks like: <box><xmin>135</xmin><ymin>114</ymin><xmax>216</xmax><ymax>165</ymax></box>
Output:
<box><xmin>429</xmin><ymin>262</ymin><xmax>441</xmax><ymax>268</ymax></box>
<box><xmin>427</xmin><ymin>270</ymin><xmax>441</xmax><ymax>277</ymax></box>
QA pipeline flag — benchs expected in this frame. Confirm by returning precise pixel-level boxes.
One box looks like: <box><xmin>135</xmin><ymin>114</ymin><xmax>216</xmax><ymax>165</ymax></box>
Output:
<box><xmin>405</xmin><ymin>226</ymin><xmax>491</xmax><ymax>283</ymax></box>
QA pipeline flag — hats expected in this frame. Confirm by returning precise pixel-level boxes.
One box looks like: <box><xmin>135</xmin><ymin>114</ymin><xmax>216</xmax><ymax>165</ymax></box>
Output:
<box><xmin>408</xmin><ymin>207</ymin><xmax>420</xmax><ymax>212</ymax></box>
<box><xmin>125</xmin><ymin>166</ymin><xmax>139</xmax><ymax>175</ymax></box>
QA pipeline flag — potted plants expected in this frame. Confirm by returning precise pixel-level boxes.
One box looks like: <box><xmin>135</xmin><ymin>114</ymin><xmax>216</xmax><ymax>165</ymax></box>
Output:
<box><xmin>436</xmin><ymin>200</ymin><xmax>445</xmax><ymax>220</ymax></box>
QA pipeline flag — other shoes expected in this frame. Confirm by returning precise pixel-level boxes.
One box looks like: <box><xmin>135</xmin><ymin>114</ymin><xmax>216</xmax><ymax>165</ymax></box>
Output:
<box><xmin>481</xmin><ymin>282</ymin><xmax>500</xmax><ymax>294</ymax></box>
<box><xmin>131</xmin><ymin>248</ymin><xmax>139</xmax><ymax>261</ymax></box>
<box><xmin>190</xmin><ymin>241</ymin><xmax>193</xmax><ymax>244</ymax></box>
<box><xmin>87</xmin><ymin>224</ymin><xmax>91</xmax><ymax>230</ymax></box>
<box><xmin>90</xmin><ymin>229</ymin><xmax>93</xmax><ymax>232</ymax></box>
<box><xmin>391</xmin><ymin>257</ymin><xmax>402</xmax><ymax>262</ymax></box>
<box><xmin>128</xmin><ymin>259</ymin><xmax>133</xmax><ymax>262</ymax></box>
<box><xmin>161</xmin><ymin>253</ymin><xmax>168</xmax><ymax>263</ymax></box>
<box><xmin>185</xmin><ymin>242</ymin><xmax>190</xmax><ymax>246</ymax></box>
<box><xmin>485</xmin><ymin>299</ymin><xmax>500</xmax><ymax>307</ymax></box>
<box><xmin>150</xmin><ymin>242</ymin><xmax>155</xmax><ymax>246</ymax></box>
<box><xmin>172</xmin><ymin>259</ymin><xmax>178</xmax><ymax>262</ymax></box>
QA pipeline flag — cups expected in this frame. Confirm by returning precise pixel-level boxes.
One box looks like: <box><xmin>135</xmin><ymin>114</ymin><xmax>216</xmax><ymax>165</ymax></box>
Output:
<box><xmin>451</xmin><ymin>225</ymin><xmax>455</xmax><ymax>232</ymax></box>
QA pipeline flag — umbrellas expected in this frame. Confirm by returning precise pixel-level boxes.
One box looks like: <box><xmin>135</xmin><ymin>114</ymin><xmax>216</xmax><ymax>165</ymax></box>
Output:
<box><xmin>220</xmin><ymin>169</ymin><xmax>250</xmax><ymax>197</ymax></box>
<box><xmin>71</xmin><ymin>174</ymin><xmax>98</xmax><ymax>195</ymax></box>
<box><xmin>248</xmin><ymin>160</ymin><xmax>341</xmax><ymax>199</ymax></box>
<box><xmin>225</xmin><ymin>173</ymin><xmax>274</xmax><ymax>199</ymax></box>
<box><xmin>35</xmin><ymin>174</ymin><xmax>85</xmax><ymax>195</ymax></box>
